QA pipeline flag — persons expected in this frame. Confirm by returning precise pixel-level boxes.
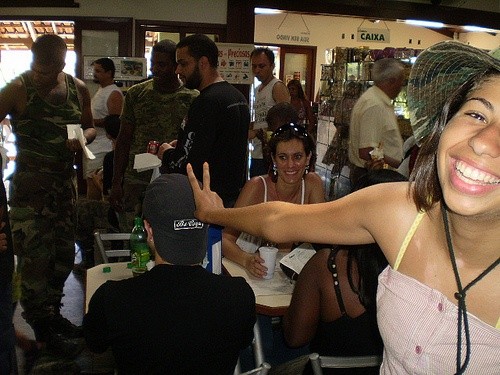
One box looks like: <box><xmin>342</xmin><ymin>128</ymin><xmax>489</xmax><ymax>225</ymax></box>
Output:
<box><xmin>248</xmin><ymin>47</ymin><xmax>291</xmax><ymax>179</ymax></box>
<box><xmin>222</xmin><ymin>124</ymin><xmax>326</xmax><ymax>279</ymax></box>
<box><xmin>282</xmin><ymin>169</ymin><xmax>410</xmax><ymax>375</ymax></box>
<box><xmin>348</xmin><ymin>59</ymin><xmax>405</xmax><ymax>193</ymax></box>
<box><xmin>112</xmin><ymin>39</ymin><xmax>200</xmax><ymax>263</ymax></box>
<box><xmin>82</xmin><ymin>172</ymin><xmax>257</xmax><ymax>375</ymax></box>
<box><xmin>0</xmin><ymin>34</ymin><xmax>97</xmax><ymax>360</ymax></box>
<box><xmin>256</xmin><ymin>102</ymin><xmax>300</xmax><ymax>174</ymax></box>
<box><xmin>287</xmin><ymin>79</ymin><xmax>318</xmax><ymax>136</ymax></box>
<box><xmin>321</xmin><ymin>82</ymin><xmax>363</xmax><ymax>200</ymax></box>
<box><xmin>0</xmin><ymin>154</ymin><xmax>41</xmax><ymax>375</ymax></box>
<box><xmin>73</xmin><ymin>115</ymin><xmax>122</xmax><ymax>279</ymax></box>
<box><xmin>187</xmin><ymin>39</ymin><xmax>500</xmax><ymax>375</ymax></box>
<box><xmin>158</xmin><ymin>35</ymin><xmax>251</xmax><ymax>275</ymax></box>
<box><xmin>85</xmin><ymin>57</ymin><xmax>125</xmax><ymax>202</ymax></box>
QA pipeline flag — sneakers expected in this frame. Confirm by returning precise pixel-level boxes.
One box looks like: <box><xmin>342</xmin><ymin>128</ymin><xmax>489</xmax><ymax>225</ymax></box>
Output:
<box><xmin>21</xmin><ymin>311</ymin><xmax>86</xmax><ymax>360</ymax></box>
<box><xmin>47</xmin><ymin>293</ymin><xmax>85</xmax><ymax>338</ymax></box>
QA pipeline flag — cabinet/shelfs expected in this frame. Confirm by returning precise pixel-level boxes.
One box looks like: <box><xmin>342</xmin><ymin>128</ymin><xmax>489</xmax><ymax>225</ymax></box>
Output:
<box><xmin>315</xmin><ymin>47</ymin><xmax>425</xmax><ymax>179</ymax></box>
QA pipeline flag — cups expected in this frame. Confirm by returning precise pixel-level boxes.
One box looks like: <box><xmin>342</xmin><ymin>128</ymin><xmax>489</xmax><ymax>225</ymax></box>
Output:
<box><xmin>66</xmin><ymin>124</ymin><xmax>81</xmax><ymax>139</ymax></box>
<box><xmin>259</xmin><ymin>247</ymin><xmax>278</xmax><ymax>279</ymax></box>
<box><xmin>370</xmin><ymin>149</ymin><xmax>384</xmax><ymax>162</ymax></box>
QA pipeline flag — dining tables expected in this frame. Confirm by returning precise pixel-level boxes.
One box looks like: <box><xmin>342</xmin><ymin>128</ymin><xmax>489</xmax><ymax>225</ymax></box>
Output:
<box><xmin>86</xmin><ymin>262</ymin><xmax>134</xmax><ymax>314</ymax></box>
<box><xmin>222</xmin><ymin>252</ymin><xmax>297</xmax><ymax>375</ymax></box>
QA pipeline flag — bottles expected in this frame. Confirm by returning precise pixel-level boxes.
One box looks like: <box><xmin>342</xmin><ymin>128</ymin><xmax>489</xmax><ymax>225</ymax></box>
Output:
<box><xmin>131</xmin><ymin>215</ymin><xmax>151</xmax><ymax>277</ymax></box>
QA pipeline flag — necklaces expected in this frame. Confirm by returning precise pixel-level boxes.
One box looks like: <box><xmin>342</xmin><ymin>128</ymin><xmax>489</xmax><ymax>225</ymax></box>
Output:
<box><xmin>275</xmin><ymin>181</ymin><xmax>301</xmax><ymax>202</ymax></box>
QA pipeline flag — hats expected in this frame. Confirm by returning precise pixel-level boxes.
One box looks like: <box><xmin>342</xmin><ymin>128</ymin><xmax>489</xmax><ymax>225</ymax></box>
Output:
<box><xmin>142</xmin><ymin>173</ymin><xmax>208</xmax><ymax>265</ymax></box>
<box><xmin>406</xmin><ymin>40</ymin><xmax>500</xmax><ymax>148</ymax></box>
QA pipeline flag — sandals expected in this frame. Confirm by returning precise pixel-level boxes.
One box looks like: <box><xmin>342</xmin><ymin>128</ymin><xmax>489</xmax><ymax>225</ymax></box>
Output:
<box><xmin>23</xmin><ymin>340</ymin><xmax>43</xmax><ymax>374</ymax></box>
